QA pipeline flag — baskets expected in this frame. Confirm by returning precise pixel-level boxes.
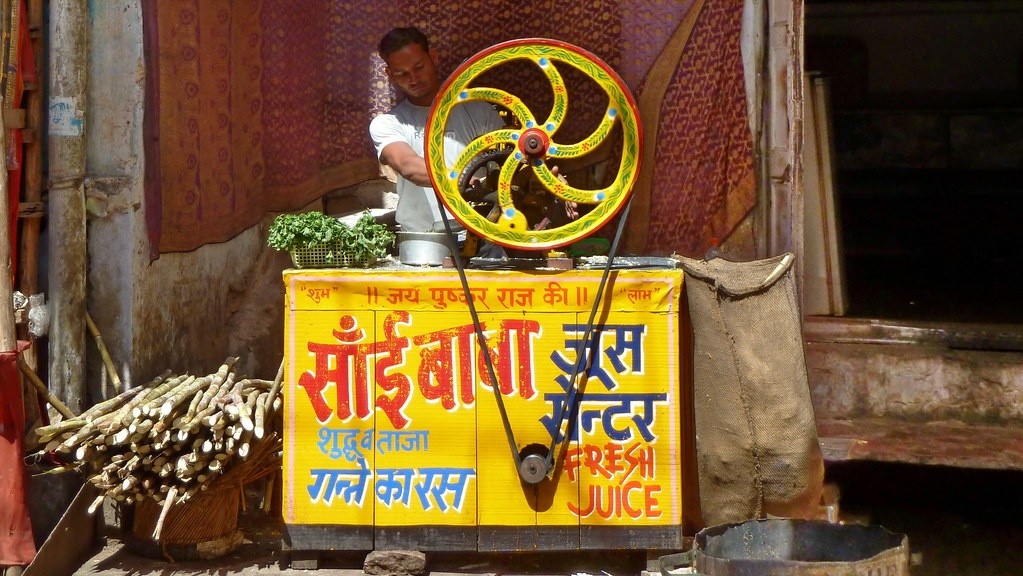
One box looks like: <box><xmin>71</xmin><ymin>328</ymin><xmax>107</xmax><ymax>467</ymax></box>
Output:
<box><xmin>286</xmin><ymin>231</ymin><xmax>378</xmax><ymax>269</ymax></box>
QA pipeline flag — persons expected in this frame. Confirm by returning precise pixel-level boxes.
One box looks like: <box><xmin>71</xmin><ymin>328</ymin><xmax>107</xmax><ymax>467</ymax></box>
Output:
<box><xmin>368</xmin><ymin>26</ymin><xmax>579</xmax><ymax>265</ymax></box>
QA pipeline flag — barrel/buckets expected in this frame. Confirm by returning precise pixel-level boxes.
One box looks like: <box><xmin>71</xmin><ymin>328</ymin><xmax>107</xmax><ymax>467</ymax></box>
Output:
<box><xmin>658</xmin><ymin>518</ymin><xmax>924</xmax><ymax>576</ymax></box>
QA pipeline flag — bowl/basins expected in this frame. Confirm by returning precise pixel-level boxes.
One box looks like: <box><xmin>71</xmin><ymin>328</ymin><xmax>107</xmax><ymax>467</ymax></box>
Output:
<box><xmin>394</xmin><ymin>231</ymin><xmax>458</xmax><ymax>265</ymax></box>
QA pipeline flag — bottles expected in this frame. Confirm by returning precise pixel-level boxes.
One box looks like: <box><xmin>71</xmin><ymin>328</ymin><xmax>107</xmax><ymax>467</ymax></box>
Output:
<box><xmin>705</xmin><ymin>235</ymin><xmax>725</xmax><ymax>262</ymax></box>
